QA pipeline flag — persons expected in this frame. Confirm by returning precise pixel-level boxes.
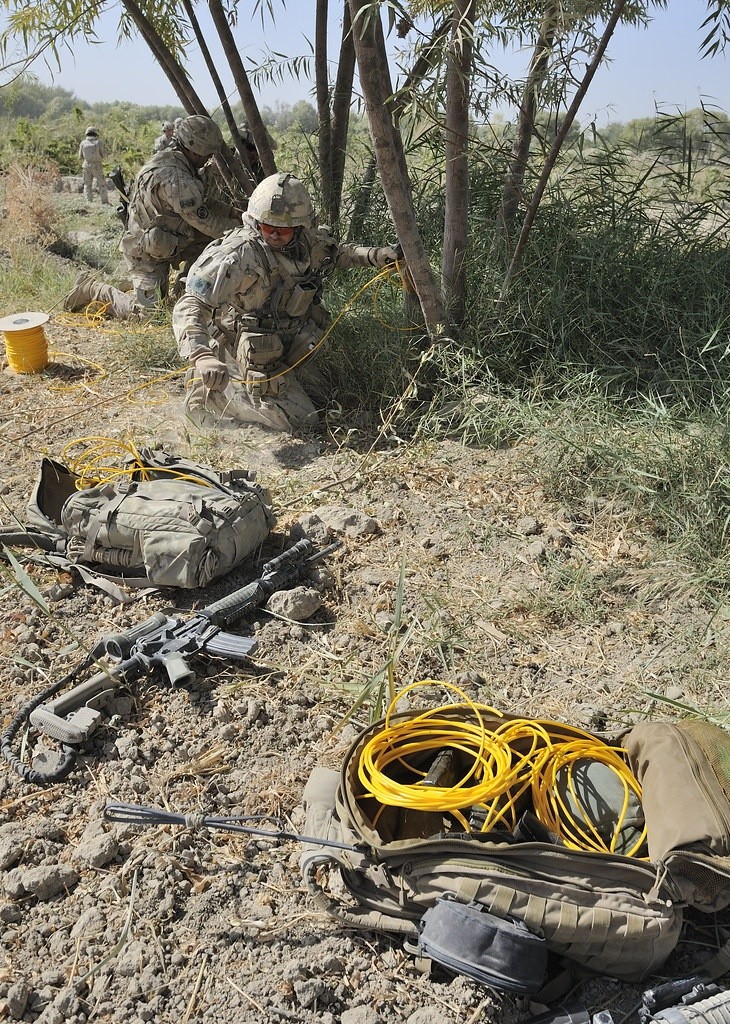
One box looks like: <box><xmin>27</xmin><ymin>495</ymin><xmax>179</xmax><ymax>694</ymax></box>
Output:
<box><xmin>154</xmin><ymin>117</ymin><xmax>183</xmax><ymax>153</ymax></box>
<box><xmin>79</xmin><ymin>126</ymin><xmax>114</xmax><ymax>207</ymax></box>
<box><xmin>234</xmin><ymin>118</ymin><xmax>279</xmax><ymax>180</ymax></box>
<box><xmin>171</xmin><ymin>173</ymin><xmax>406</xmax><ymax>433</ymax></box>
<box><xmin>63</xmin><ymin>115</ymin><xmax>243</xmax><ymax>323</ymax></box>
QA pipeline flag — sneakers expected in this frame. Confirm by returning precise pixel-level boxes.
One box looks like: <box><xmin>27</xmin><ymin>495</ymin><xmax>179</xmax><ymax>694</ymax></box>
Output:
<box><xmin>184</xmin><ymin>367</ymin><xmax>216</xmax><ymax>431</ymax></box>
<box><xmin>64</xmin><ymin>271</ymin><xmax>96</xmax><ymax>312</ymax></box>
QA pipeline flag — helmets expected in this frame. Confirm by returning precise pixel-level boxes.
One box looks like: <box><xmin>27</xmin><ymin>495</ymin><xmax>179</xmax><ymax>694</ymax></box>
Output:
<box><xmin>237</xmin><ymin>120</ymin><xmax>278</xmax><ymax>149</ymax></box>
<box><xmin>177</xmin><ymin>115</ymin><xmax>223</xmax><ymax>156</ymax></box>
<box><xmin>247</xmin><ymin>171</ymin><xmax>315</xmax><ymax>227</ymax></box>
<box><xmin>174</xmin><ymin>118</ymin><xmax>183</xmax><ymax>126</ymax></box>
<box><xmin>161</xmin><ymin>122</ymin><xmax>175</xmax><ymax>130</ymax></box>
<box><xmin>86</xmin><ymin>126</ymin><xmax>98</xmax><ymax>136</ymax></box>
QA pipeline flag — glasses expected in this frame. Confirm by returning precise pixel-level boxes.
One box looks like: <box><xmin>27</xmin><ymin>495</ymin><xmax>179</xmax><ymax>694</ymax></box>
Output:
<box><xmin>258</xmin><ymin>222</ymin><xmax>297</xmax><ymax>236</ymax></box>
<box><xmin>241</xmin><ymin>139</ymin><xmax>257</xmax><ymax>152</ymax></box>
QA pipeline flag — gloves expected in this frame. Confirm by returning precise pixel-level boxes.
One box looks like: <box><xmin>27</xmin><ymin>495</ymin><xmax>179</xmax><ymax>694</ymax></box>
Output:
<box><xmin>195</xmin><ymin>356</ymin><xmax>229</xmax><ymax>392</ymax></box>
<box><xmin>369</xmin><ymin>247</ymin><xmax>398</xmax><ymax>269</ymax></box>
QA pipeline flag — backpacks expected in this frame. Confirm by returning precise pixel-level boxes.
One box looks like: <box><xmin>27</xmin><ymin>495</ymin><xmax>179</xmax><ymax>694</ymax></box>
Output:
<box><xmin>0</xmin><ymin>446</ymin><xmax>279</xmax><ymax>603</ymax></box>
<box><xmin>299</xmin><ymin>708</ymin><xmax>730</xmax><ymax>995</ymax></box>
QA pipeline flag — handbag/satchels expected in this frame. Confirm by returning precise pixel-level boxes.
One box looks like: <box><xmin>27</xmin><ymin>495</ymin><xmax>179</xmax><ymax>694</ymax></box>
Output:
<box><xmin>235</xmin><ymin>302</ymin><xmax>331</xmax><ymax>408</ymax></box>
<box><xmin>143</xmin><ymin>215</ymin><xmax>181</xmax><ymax>260</ymax></box>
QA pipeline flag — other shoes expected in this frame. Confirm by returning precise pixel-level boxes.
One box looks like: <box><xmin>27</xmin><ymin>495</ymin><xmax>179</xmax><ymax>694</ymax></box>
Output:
<box><xmin>103</xmin><ymin>203</ymin><xmax>113</xmax><ymax>207</ymax></box>
